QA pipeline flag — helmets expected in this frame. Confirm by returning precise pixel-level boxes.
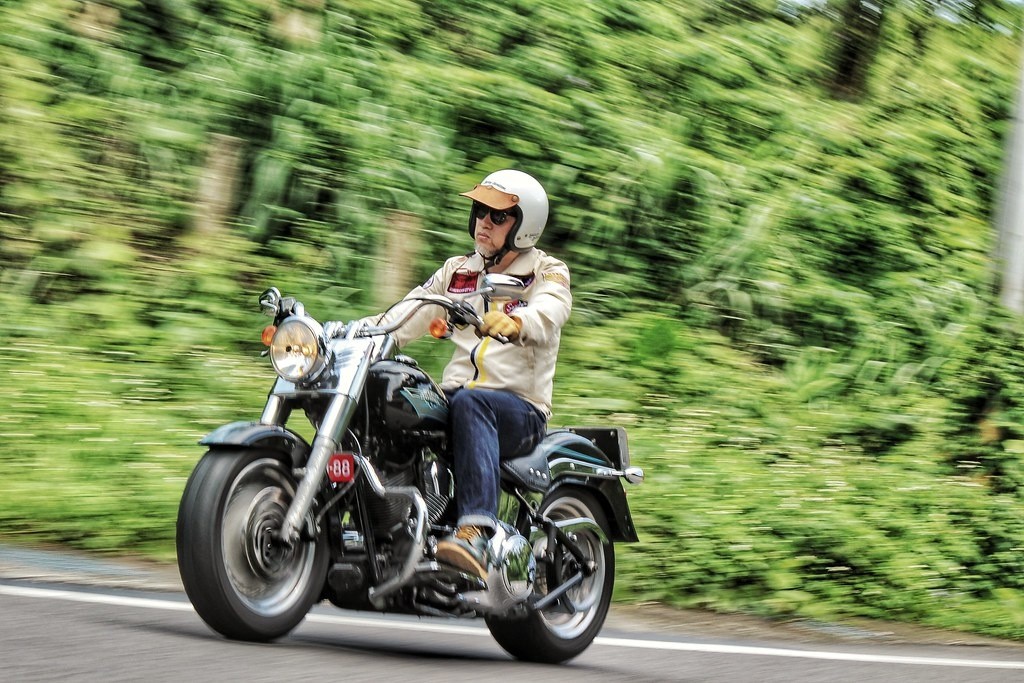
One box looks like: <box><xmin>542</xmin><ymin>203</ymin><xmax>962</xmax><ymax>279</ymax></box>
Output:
<box><xmin>459</xmin><ymin>169</ymin><xmax>549</xmax><ymax>251</ymax></box>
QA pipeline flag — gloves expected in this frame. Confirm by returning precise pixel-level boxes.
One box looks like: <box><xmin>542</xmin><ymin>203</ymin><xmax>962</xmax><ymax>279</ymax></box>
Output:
<box><xmin>476</xmin><ymin>310</ymin><xmax>521</xmax><ymax>344</ymax></box>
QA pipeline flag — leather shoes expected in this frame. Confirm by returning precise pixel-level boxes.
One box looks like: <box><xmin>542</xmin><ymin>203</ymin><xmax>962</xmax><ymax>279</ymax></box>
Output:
<box><xmin>436</xmin><ymin>517</ymin><xmax>495</xmax><ymax>580</ymax></box>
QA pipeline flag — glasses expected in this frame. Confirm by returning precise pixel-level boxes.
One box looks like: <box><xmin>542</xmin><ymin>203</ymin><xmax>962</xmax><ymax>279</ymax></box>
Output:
<box><xmin>469</xmin><ymin>202</ymin><xmax>515</xmax><ymax>225</ymax></box>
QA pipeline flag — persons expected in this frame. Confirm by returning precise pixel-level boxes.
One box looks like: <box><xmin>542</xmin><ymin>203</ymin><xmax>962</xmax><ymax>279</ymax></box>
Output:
<box><xmin>318</xmin><ymin>170</ymin><xmax>573</xmax><ymax>580</ymax></box>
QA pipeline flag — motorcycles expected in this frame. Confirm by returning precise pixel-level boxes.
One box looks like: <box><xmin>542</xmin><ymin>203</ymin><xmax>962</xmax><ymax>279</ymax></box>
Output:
<box><xmin>175</xmin><ymin>271</ymin><xmax>639</xmax><ymax>664</ymax></box>
